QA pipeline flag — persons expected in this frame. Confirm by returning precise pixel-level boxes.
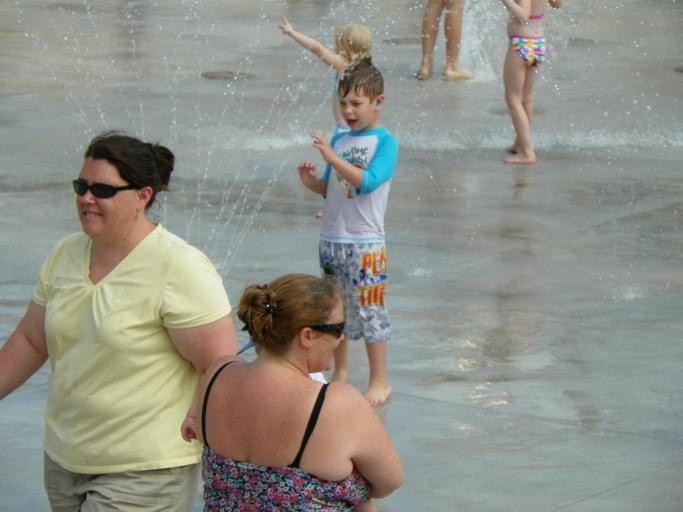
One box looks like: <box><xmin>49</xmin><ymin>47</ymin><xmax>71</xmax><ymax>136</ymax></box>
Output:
<box><xmin>279</xmin><ymin>14</ymin><xmax>372</xmax><ymax>218</ymax></box>
<box><xmin>182</xmin><ymin>334</ymin><xmax>378</xmax><ymax>512</ymax></box>
<box><xmin>193</xmin><ymin>271</ymin><xmax>404</xmax><ymax>512</ymax></box>
<box><xmin>415</xmin><ymin>0</ymin><xmax>469</xmax><ymax>80</ymax></box>
<box><xmin>0</xmin><ymin>129</ymin><xmax>240</xmax><ymax>512</ymax></box>
<box><xmin>296</xmin><ymin>58</ymin><xmax>398</xmax><ymax>407</ymax></box>
<box><xmin>500</xmin><ymin>0</ymin><xmax>563</xmax><ymax>163</ymax></box>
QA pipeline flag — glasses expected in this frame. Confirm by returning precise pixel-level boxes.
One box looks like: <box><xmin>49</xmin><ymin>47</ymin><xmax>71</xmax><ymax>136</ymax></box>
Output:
<box><xmin>312</xmin><ymin>321</ymin><xmax>345</xmax><ymax>338</ymax></box>
<box><xmin>73</xmin><ymin>179</ymin><xmax>134</xmax><ymax>198</ymax></box>
